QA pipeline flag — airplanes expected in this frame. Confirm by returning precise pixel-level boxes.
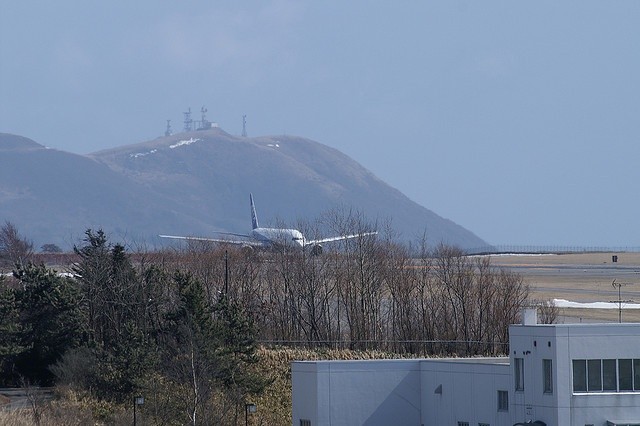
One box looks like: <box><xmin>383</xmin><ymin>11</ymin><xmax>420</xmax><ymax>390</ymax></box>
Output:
<box><xmin>157</xmin><ymin>193</ymin><xmax>379</xmax><ymax>257</ymax></box>
